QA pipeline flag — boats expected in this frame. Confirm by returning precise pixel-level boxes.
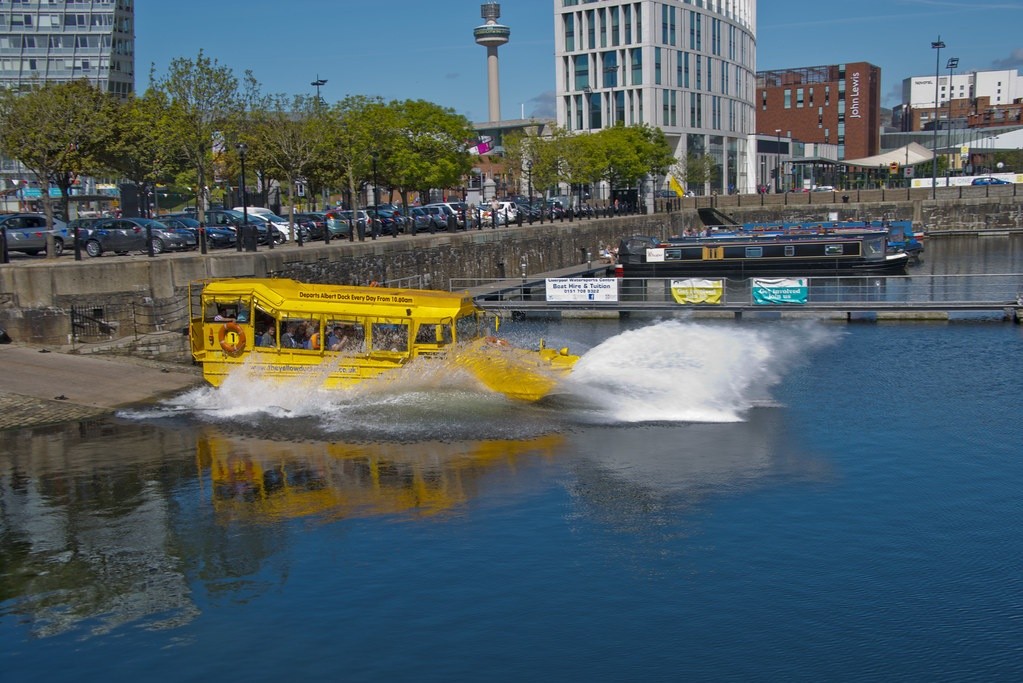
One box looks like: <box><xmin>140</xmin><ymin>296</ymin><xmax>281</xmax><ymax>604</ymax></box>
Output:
<box><xmin>614</xmin><ymin>221</ymin><xmax>926</xmax><ymax>276</ymax></box>
<box><xmin>182</xmin><ymin>274</ymin><xmax>579</xmax><ymax>407</ymax></box>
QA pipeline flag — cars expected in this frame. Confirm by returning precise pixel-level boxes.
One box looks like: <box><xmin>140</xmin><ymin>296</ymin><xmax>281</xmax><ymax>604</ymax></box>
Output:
<box><xmin>971</xmin><ymin>177</ymin><xmax>1014</xmax><ymax>185</ymax></box>
<box><xmin>817</xmin><ymin>186</ymin><xmax>839</xmax><ymax>193</ymax></box>
<box><xmin>68</xmin><ymin>195</ymin><xmax>595</xmax><ymax>258</ymax></box>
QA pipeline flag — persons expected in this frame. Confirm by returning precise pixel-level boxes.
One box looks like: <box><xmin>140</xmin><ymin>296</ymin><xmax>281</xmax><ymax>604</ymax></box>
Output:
<box><xmin>688</xmin><ymin>181</ymin><xmax>771</xmax><ymax>196</ymax></box>
<box><xmin>466</xmin><ymin>196</ymin><xmax>500</xmax><ymax>229</ymax></box>
<box><xmin>605</xmin><ymin>227</ymin><xmax>711</xmax><ymax>267</ymax></box>
<box><xmin>212</xmin><ymin>306</ymin><xmax>451</xmax><ymax>352</ymax></box>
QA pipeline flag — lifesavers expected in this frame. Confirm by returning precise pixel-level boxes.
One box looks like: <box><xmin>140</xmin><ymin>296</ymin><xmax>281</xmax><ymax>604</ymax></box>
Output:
<box><xmin>370</xmin><ymin>281</ymin><xmax>380</xmax><ymax>288</ymax></box>
<box><xmin>485</xmin><ymin>337</ymin><xmax>511</xmax><ymax>350</ymax></box>
<box><xmin>218</xmin><ymin>323</ymin><xmax>246</xmax><ymax>353</ymax></box>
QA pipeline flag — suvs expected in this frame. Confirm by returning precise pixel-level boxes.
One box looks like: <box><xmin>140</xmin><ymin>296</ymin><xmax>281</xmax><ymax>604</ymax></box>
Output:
<box><xmin>0</xmin><ymin>212</ymin><xmax>70</xmax><ymax>256</ymax></box>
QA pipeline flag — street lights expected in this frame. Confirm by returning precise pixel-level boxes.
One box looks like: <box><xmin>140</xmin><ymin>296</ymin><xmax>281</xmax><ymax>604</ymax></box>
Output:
<box><xmin>775</xmin><ymin>129</ymin><xmax>782</xmax><ymax>194</ymax></box>
<box><xmin>238</xmin><ymin>142</ymin><xmax>257</xmax><ymax>252</ymax></box>
<box><xmin>946</xmin><ymin>58</ymin><xmax>960</xmax><ymax>188</ymax></box>
<box><xmin>930</xmin><ymin>35</ymin><xmax>947</xmax><ymax>199</ymax></box>
<box><xmin>526</xmin><ymin>160</ymin><xmax>534</xmax><ymax>223</ymax></box>
